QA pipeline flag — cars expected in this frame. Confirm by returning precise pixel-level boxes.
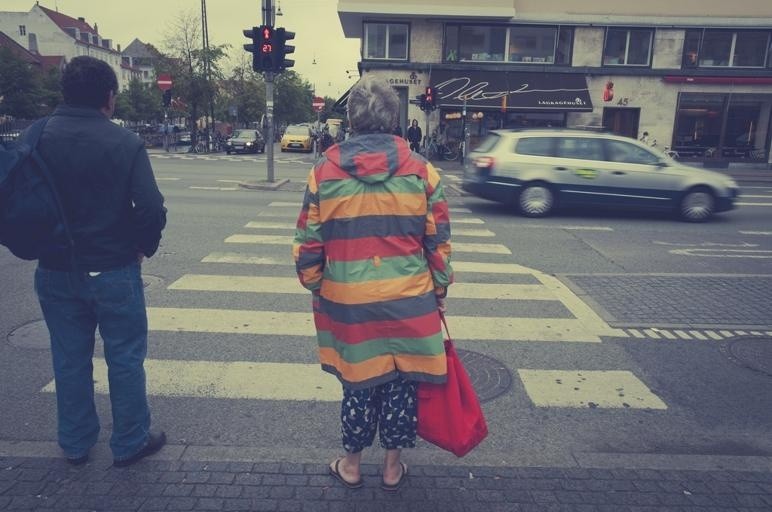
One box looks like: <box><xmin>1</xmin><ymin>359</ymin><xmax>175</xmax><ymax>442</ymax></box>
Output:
<box><xmin>280</xmin><ymin>122</ymin><xmax>314</xmax><ymax>152</ymax></box>
<box><xmin>226</xmin><ymin>130</ymin><xmax>265</xmax><ymax>154</ymax></box>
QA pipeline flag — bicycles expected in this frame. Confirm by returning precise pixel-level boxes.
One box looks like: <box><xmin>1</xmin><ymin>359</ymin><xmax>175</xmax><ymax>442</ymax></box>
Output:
<box><xmin>420</xmin><ymin>136</ymin><xmax>460</xmax><ymax>162</ymax></box>
<box><xmin>195</xmin><ymin>140</ymin><xmax>209</xmax><ymax>154</ymax></box>
<box><xmin>648</xmin><ymin>140</ymin><xmax>680</xmax><ymax>161</ymax></box>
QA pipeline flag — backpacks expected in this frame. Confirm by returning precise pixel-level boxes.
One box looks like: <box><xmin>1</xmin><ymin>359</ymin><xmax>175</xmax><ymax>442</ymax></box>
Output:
<box><xmin>1</xmin><ymin>114</ymin><xmax>76</xmax><ymax>262</ymax></box>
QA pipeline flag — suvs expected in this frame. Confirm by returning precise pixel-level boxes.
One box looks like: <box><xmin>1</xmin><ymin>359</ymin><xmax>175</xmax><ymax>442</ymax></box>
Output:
<box><xmin>461</xmin><ymin>128</ymin><xmax>739</xmax><ymax>221</ymax></box>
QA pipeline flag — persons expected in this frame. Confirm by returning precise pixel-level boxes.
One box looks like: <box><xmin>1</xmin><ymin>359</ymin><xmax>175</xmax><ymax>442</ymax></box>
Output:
<box><xmin>406</xmin><ymin>117</ymin><xmax>423</xmax><ymax>153</ymax></box>
<box><xmin>640</xmin><ymin>130</ymin><xmax>649</xmax><ymax>144</ymax></box>
<box><xmin>291</xmin><ymin>76</ymin><xmax>454</xmax><ymax>490</ymax></box>
<box><xmin>432</xmin><ymin>117</ymin><xmax>450</xmax><ymax>161</ymax></box>
<box><xmin>310</xmin><ymin>118</ymin><xmax>351</xmax><ymax>155</ymax></box>
<box><xmin>13</xmin><ymin>54</ymin><xmax>168</xmax><ymax>468</ymax></box>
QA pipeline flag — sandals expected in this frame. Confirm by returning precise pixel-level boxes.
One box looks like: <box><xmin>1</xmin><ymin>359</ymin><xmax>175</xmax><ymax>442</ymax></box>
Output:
<box><xmin>328</xmin><ymin>456</ymin><xmax>362</xmax><ymax>489</ymax></box>
<box><xmin>381</xmin><ymin>460</ymin><xmax>409</xmax><ymax>492</ymax></box>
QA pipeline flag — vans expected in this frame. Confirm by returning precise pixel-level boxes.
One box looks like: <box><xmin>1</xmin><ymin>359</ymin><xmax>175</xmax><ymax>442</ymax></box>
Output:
<box><xmin>325</xmin><ymin>119</ymin><xmax>345</xmax><ymax>140</ymax></box>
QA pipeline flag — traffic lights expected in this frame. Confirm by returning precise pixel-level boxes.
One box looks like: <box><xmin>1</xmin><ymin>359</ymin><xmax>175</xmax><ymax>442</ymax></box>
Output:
<box><xmin>425</xmin><ymin>87</ymin><xmax>436</xmax><ymax>110</ymax></box>
<box><xmin>243</xmin><ymin>25</ymin><xmax>296</xmax><ymax>70</ymax></box>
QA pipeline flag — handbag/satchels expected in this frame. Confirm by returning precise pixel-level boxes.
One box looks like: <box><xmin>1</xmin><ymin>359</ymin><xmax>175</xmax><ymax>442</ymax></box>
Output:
<box><xmin>415</xmin><ymin>309</ymin><xmax>489</xmax><ymax>459</ymax></box>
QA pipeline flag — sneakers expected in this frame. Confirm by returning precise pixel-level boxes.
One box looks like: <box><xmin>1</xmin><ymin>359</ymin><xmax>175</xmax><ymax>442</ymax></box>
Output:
<box><xmin>114</xmin><ymin>429</ymin><xmax>167</xmax><ymax>466</ymax></box>
<box><xmin>65</xmin><ymin>450</ymin><xmax>89</xmax><ymax>466</ymax></box>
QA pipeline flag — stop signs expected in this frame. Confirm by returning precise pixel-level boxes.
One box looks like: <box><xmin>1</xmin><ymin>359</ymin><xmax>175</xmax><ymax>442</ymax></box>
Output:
<box><xmin>157</xmin><ymin>76</ymin><xmax>172</xmax><ymax>91</ymax></box>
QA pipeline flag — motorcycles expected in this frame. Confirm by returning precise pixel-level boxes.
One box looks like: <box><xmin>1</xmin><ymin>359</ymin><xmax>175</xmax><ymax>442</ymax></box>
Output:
<box><xmin>214</xmin><ymin>137</ymin><xmax>225</xmax><ymax>152</ymax></box>
